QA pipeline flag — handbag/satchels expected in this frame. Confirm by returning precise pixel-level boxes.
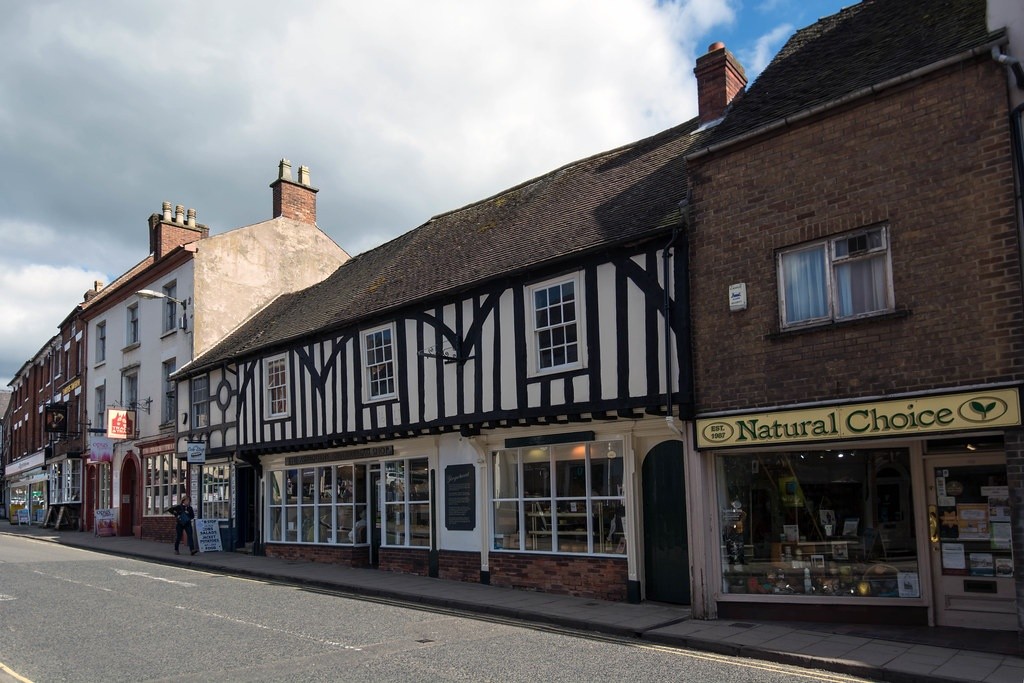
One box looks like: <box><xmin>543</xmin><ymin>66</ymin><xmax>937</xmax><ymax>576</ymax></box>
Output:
<box><xmin>175</xmin><ymin>513</ymin><xmax>188</xmax><ymax>524</ymax></box>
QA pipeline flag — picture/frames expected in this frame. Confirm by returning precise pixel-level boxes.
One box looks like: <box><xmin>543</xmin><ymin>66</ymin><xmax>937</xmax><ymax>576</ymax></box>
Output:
<box><xmin>615</xmin><ymin>537</ymin><xmax>626</xmax><ymax>554</ymax></box>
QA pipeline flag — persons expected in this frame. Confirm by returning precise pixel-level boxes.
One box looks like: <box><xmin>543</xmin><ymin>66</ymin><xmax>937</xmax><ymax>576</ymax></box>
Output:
<box><xmin>348</xmin><ymin>511</ymin><xmax>367</xmax><ymax>544</ymax></box>
<box><xmin>168</xmin><ymin>495</ymin><xmax>198</xmax><ymax>556</ymax></box>
<box><xmin>302</xmin><ymin>508</ymin><xmax>328</xmax><ymax>543</ymax></box>
<box><xmin>386</xmin><ymin>478</ymin><xmax>404</xmax><ymax>512</ymax></box>
<box><xmin>339</xmin><ymin>481</ymin><xmax>352</xmax><ymax>503</ymax></box>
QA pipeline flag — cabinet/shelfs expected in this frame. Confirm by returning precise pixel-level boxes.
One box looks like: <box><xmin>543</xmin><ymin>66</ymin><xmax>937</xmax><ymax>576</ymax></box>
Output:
<box><xmin>528</xmin><ymin>502</ymin><xmax>604</xmax><ymax>553</ymax></box>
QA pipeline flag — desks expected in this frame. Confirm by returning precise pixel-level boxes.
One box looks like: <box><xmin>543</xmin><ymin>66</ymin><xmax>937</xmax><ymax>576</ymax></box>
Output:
<box><xmin>326</xmin><ymin>529</ymin><xmax>350</xmax><ymax>532</ymax></box>
<box><xmin>286</xmin><ymin>529</ymin><xmax>297</xmax><ymax>532</ymax></box>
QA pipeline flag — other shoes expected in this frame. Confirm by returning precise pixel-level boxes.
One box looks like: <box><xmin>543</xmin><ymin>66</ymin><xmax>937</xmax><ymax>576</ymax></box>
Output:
<box><xmin>175</xmin><ymin>550</ymin><xmax>179</xmax><ymax>554</ymax></box>
<box><xmin>191</xmin><ymin>550</ymin><xmax>198</xmax><ymax>556</ymax></box>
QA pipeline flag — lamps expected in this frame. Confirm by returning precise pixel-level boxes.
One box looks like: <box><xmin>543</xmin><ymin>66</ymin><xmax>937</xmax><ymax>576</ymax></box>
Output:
<box><xmin>134</xmin><ymin>289</ymin><xmax>186</xmax><ymax>310</ymax></box>
